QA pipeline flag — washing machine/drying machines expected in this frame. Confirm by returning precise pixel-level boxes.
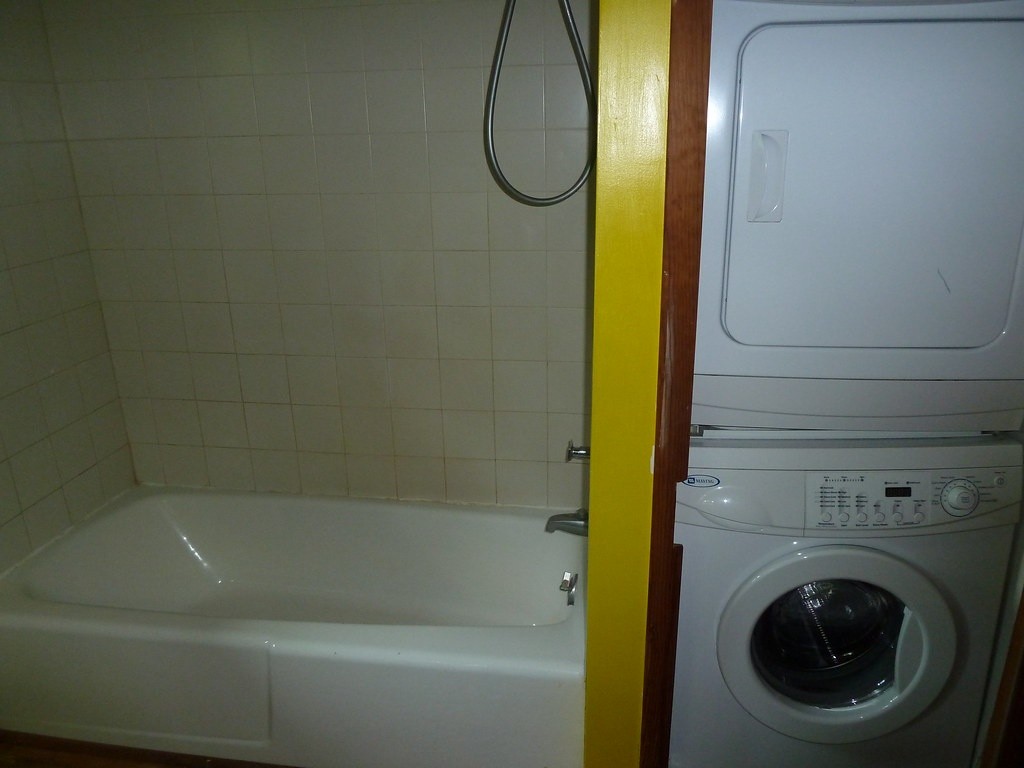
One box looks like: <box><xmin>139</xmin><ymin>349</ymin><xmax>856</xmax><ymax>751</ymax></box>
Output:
<box><xmin>665</xmin><ymin>427</ymin><xmax>1024</xmax><ymax>768</ymax></box>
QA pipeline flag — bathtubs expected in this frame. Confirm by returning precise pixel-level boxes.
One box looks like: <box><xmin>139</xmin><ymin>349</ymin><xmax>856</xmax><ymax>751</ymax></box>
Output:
<box><xmin>0</xmin><ymin>480</ymin><xmax>588</xmax><ymax>767</ymax></box>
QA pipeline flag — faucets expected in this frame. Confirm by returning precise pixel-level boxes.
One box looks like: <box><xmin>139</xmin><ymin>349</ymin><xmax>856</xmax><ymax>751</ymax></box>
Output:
<box><xmin>544</xmin><ymin>507</ymin><xmax>589</xmax><ymax>539</ymax></box>
